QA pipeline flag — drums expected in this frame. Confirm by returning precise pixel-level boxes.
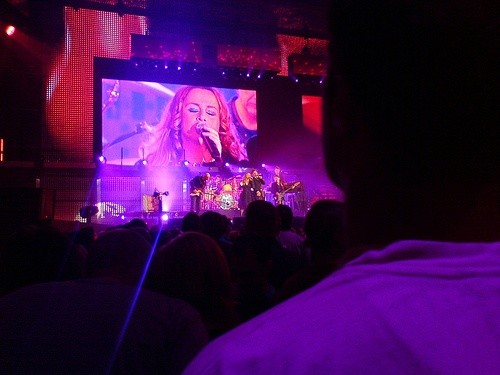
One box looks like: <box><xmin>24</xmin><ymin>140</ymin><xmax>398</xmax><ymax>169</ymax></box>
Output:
<box><xmin>219</xmin><ymin>193</ymin><xmax>235</xmax><ymax>210</ymax></box>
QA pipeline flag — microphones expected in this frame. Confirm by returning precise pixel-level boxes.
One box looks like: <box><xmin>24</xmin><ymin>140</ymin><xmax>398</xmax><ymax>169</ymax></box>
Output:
<box><xmin>195</xmin><ymin>122</ymin><xmax>220</xmax><ymax>162</ymax></box>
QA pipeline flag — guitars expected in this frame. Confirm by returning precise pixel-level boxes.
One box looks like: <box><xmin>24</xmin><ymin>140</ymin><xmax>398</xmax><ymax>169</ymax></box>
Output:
<box><xmin>274</xmin><ymin>181</ymin><xmax>301</xmax><ymax>204</ymax></box>
<box><xmin>189</xmin><ymin>190</ymin><xmax>215</xmax><ymax>197</ymax></box>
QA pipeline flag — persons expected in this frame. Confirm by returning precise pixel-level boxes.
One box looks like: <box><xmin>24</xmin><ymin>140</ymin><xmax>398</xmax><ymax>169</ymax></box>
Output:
<box><xmin>0</xmin><ymin>165</ymin><xmax>387</xmax><ymax>375</ymax></box>
<box><xmin>133</xmin><ymin>85</ymin><xmax>257</xmax><ymax>166</ymax></box>
<box><xmin>183</xmin><ymin>0</ymin><xmax>500</xmax><ymax>375</ymax></box>
